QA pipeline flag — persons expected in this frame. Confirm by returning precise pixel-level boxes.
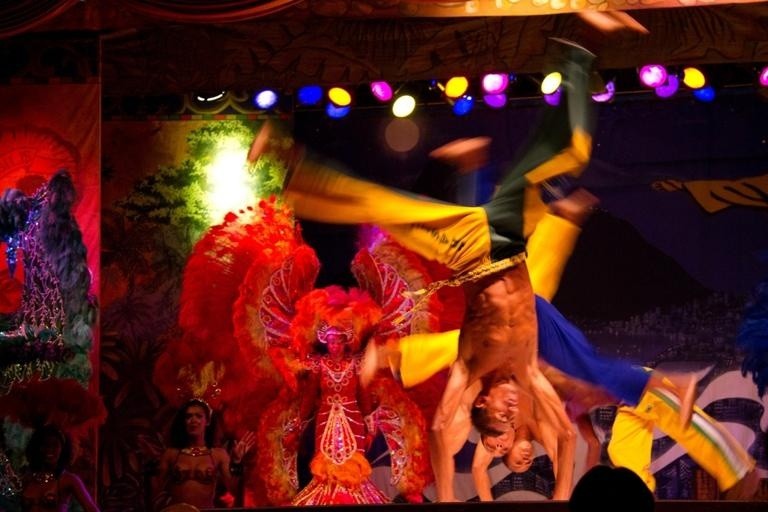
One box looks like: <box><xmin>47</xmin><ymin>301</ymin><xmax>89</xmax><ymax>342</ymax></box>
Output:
<box><xmin>135</xmin><ymin>398</ymin><xmax>256</xmax><ymax>511</ymax></box>
<box><xmin>17</xmin><ymin>426</ymin><xmax>99</xmax><ymax>512</ymax></box>
<box><xmin>245</xmin><ymin>38</ymin><xmax>768</xmax><ymax>511</ymax></box>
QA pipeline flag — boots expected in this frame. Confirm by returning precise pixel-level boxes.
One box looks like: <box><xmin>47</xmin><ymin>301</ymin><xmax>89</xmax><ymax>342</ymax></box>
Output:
<box><xmin>247</xmin><ymin>118</ymin><xmax>304</xmax><ymax>171</ymax></box>
